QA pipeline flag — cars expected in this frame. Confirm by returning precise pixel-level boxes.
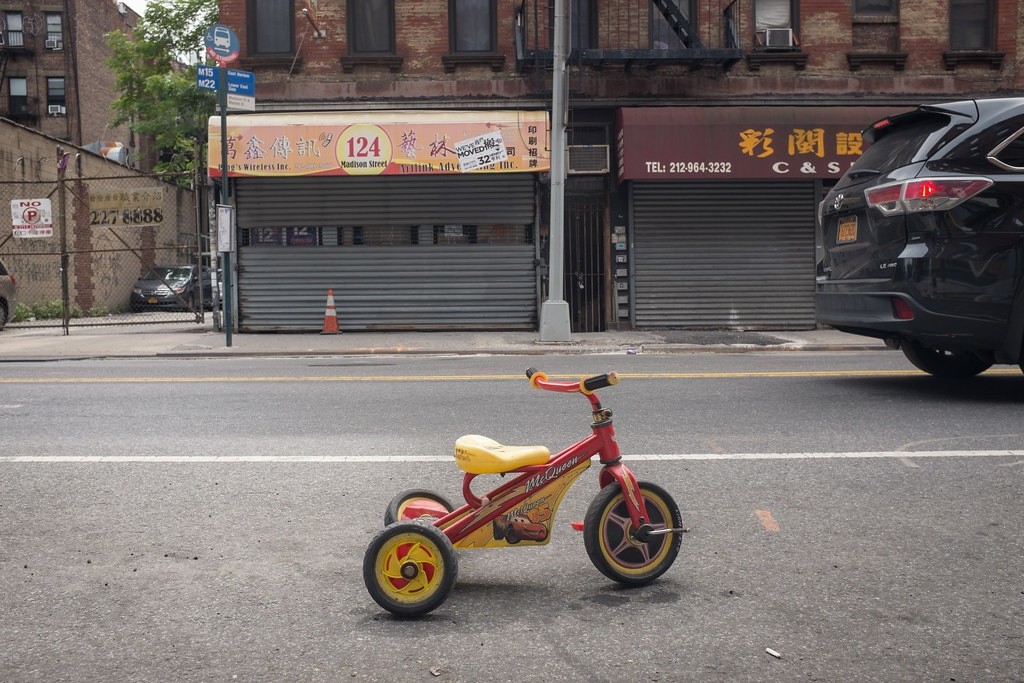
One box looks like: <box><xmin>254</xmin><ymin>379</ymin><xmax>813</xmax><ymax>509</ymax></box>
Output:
<box><xmin>0</xmin><ymin>260</ymin><xmax>18</xmax><ymax>331</ymax></box>
<box><xmin>131</xmin><ymin>264</ymin><xmax>222</xmax><ymax>312</ymax></box>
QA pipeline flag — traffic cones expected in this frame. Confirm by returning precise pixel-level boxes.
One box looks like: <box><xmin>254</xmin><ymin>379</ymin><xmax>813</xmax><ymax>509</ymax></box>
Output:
<box><xmin>320</xmin><ymin>290</ymin><xmax>342</xmax><ymax>334</ymax></box>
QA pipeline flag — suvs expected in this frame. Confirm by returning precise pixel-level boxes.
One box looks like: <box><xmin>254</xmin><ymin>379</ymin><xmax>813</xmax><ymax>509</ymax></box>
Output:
<box><xmin>815</xmin><ymin>97</ymin><xmax>1024</xmax><ymax>376</ymax></box>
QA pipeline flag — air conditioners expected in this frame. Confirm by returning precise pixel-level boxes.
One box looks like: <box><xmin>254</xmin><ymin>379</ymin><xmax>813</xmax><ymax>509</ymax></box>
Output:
<box><xmin>47</xmin><ymin>105</ymin><xmax>59</xmax><ymax>113</ymax></box>
<box><xmin>567</xmin><ymin>147</ymin><xmax>610</xmax><ymax>173</ymax></box>
<box><xmin>46</xmin><ymin>41</ymin><xmax>57</xmax><ymax>48</ymax></box>
<box><xmin>764</xmin><ymin>28</ymin><xmax>793</xmax><ymax>46</ymax></box>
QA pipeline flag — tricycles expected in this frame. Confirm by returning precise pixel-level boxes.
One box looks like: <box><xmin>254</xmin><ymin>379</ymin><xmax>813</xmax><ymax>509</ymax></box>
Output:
<box><xmin>364</xmin><ymin>369</ymin><xmax>691</xmax><ymax>617</ymax></box>
